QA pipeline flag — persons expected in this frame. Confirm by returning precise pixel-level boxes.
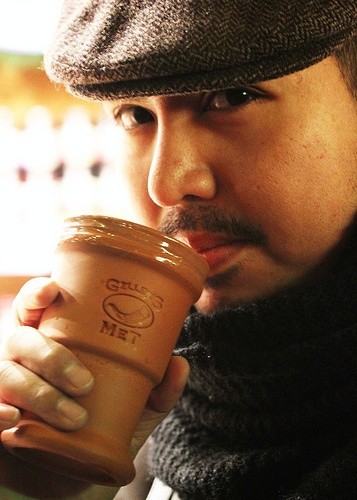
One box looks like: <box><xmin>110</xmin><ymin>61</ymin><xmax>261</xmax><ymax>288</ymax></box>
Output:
<box><xmin>1</xmin><ymin>1</ymin><xmax>357</xmax><ymax>500</ymax></box>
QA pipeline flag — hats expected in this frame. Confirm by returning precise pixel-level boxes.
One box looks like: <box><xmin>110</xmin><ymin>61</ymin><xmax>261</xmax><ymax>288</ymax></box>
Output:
<box><xmin>42</xmin><ymin>0</ymin><xmax>356</xmax><ymax>101</ymax></box>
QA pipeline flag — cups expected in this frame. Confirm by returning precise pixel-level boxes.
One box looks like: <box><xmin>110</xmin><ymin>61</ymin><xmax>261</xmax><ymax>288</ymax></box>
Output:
<box><xmin>2</xmin><ymin>214</ymin><xmax>210</xmax><ymax>487</ymax></box>
